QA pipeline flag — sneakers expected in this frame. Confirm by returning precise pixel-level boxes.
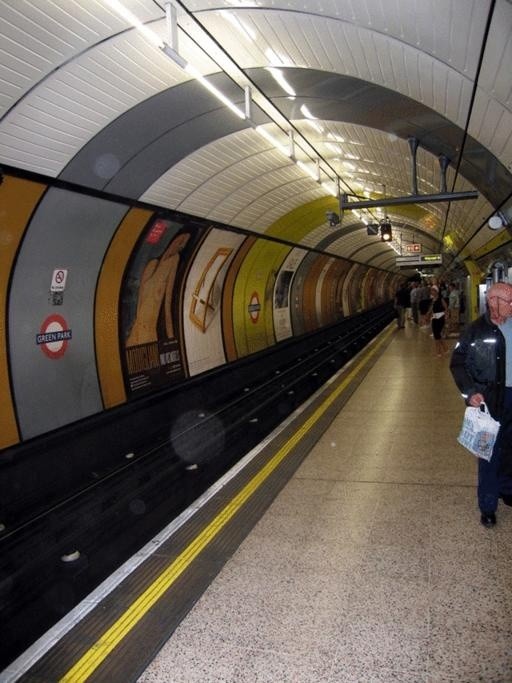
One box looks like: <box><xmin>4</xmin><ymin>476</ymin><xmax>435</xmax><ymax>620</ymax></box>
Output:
<box><xmin>480</xmin><ymin>513</ymin><xmax>495</xmax><ymax>527</ymax></box>
<box><xmin>502</xmin><ymin>494</ymin><xmax>512</xmax><ymax>507</ymax></box>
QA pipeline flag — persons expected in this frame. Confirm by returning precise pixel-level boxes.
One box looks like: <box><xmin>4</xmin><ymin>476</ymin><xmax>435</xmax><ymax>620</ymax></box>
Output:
<box><xmin>396</xmin><ymin>278</ymin><xmax>466</xmax><ymax>357</ymax></box>
<box><xmin>450</xmin><ymin>283</ymin><xmax>512</xmax><ymax>527</ymax></box>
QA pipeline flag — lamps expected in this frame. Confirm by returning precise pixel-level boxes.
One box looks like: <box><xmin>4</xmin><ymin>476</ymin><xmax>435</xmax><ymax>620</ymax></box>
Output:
<box><xmin>380</xmin><ymin>203</ymin><xmax>392</xmax><ymax>242</ymax></box>
